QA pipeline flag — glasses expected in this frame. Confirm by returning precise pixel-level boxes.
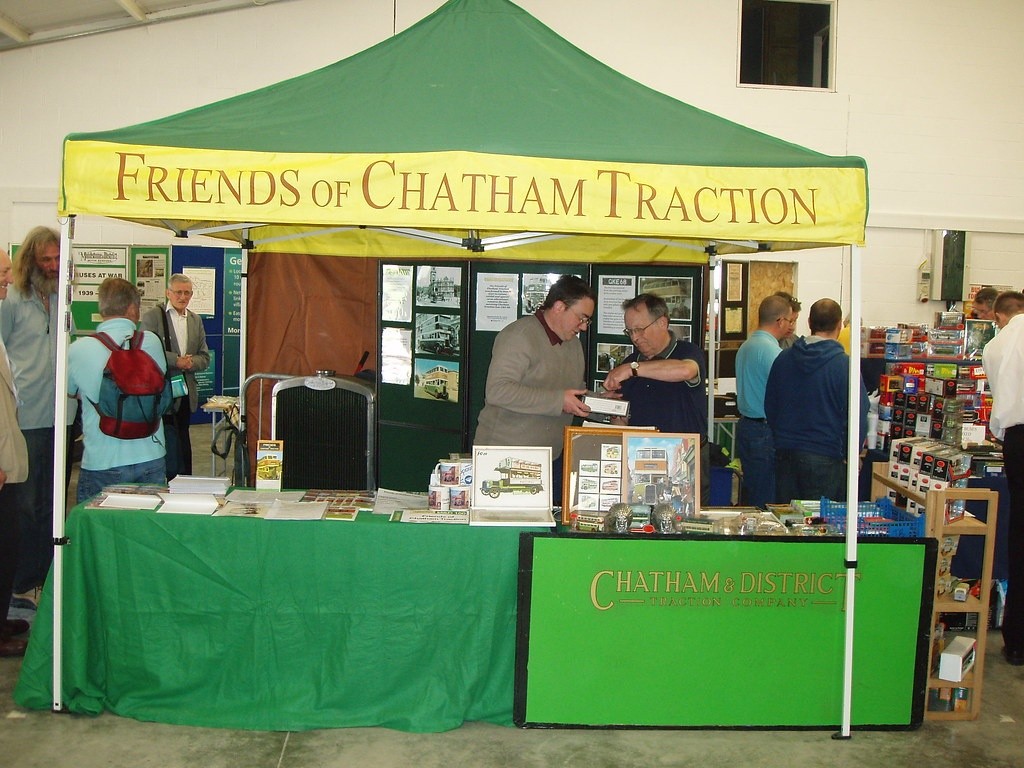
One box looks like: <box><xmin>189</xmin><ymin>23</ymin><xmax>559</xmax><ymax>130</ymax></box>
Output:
<box><xmin>562</xmin><ymin>299</ymin><xmax>592</xmax><ymax>326</ymax></box>
<box><xmin>170</xmin><ymin>289</ymin><xmax>192</xmax><ymax>297</ymax></box>
<box><xmin>972</xmin><ymin>307</ymin><xmax>991</xmax><ymax>315</ymax></box>
<box><xmin>788</xmin><ymin>298</ymin><xmax>798</xmax><ymax>303</ymax></box>
<box><xmin>623</xmin><ymin>315</ymin><xmax>662</xmax><ymax>336</ymax></box>
<box><xmin>777</xmin><ymin>317</ymin><xmax>795</xmax><ymax>326</ymax></box>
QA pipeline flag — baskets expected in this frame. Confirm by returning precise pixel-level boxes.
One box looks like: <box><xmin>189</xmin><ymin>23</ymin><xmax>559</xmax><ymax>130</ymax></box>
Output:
<box><xmin>821</xmin><ymin>494</ymin><xmax>926</xmax><ymax>538</ymax></box>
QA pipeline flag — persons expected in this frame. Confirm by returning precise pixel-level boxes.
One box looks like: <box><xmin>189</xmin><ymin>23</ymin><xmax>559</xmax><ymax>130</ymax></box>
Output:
<box><xmin>0</xmin><ymin>225</ymin><xmax>78</xmax><ymax>611</ymax></box>
<box><xmin>836</xmin><ymin>312</ymin><xmax>863</xmax><ymax>356</ymax></box>
<box><xmin>67</xmin><ymin>277</ymin><xmax>167</xmax><ymax>505</ymax></box>
<box><xmin>735</xmin><ymin>296</ymin><xmax>794</xmax><ymax>508</ymax></box>
<box><xmin>139</xmin><ymin>273</ymin><xmax>210</xmax><ymax>483</ymax></box>
<box><xmin>773</xmin><ymin>291</ymin><xmax>801</xmax><ymax>349</ymax></box>
<box><xmin>982</xmin><ymin>291</ymin><xmax>1024</xmax><ymax>666</ymax></box>
<box><xmin>474</xmin><ymin>275</ymin><xmax>623</xmax><ymax>506</ymax></box>
<box><xmin>603</xmin><ymin>293</ymin><xmax>711</xmax><ymax>506</ymax></box>
<box><xmin>0</xmin><ymin>246</ymin><xmax>30</xmax><ymax>644</ymax></box>
<box><xmin>764</xmin><ymin>298</ymin><xmax>870</xmax><ymax>503</ymax></box>
<box><xmin>974</xmin><ymin>287</ymin><xmax>998</xmax><ymax>320</ymax></box>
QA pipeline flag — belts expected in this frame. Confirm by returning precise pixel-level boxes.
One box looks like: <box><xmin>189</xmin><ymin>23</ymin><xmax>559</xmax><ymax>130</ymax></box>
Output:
<box><xmin>740</xmin><ymin>414</ymin><xmax>767</xmax><ymax>423</ymax></box>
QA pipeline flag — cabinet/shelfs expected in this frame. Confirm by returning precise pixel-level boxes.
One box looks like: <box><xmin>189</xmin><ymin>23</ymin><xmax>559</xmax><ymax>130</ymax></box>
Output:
<box><xmin>870</xmin><ymin>463</ymin><xmax>999</xmax><ymax>725</ymax></box>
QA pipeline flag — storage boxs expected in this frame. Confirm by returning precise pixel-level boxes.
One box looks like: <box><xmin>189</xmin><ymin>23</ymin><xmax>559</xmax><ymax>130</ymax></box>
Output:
<box><xmin>821</xmin><ymin>496</ymin><xmax>926</xmax><ymax>538</ymax></box>
<box><xmin>861</xmin><ymin>310</ymin><xmax>1007</xmax><ymax>682</ymax></box>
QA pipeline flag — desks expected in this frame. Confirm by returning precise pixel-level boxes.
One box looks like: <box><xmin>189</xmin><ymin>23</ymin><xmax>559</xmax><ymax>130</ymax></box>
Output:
<box><xmin>13</xmin><ymin>483</ymin><xmax>925</xmax><ymax>728</ymax></box>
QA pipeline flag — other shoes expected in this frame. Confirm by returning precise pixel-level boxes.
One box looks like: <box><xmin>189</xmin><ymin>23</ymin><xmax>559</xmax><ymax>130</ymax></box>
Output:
<box><xmin>0</xmin><ymin>637</ymin><xmax>27</xmax><ymax>656</ymax></box>
<box><xmin>8</xmin><ymin>618</ymin><xmax>30</xmax><ymax>636</ymax></box>
<box><xmin>1002</xmin><ymin>645</ymin><xmax>1024</xmax><ymax>666</ymax></box>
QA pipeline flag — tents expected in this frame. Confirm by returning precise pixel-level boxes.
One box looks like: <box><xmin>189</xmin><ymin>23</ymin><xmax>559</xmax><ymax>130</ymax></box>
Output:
<box><xmin>52</xmin><ymin>0</ymin><xmax>869</xmax><ymax>737</ymax></box>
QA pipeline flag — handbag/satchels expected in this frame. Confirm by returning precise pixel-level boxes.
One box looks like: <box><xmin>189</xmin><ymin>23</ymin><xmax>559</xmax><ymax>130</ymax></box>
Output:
<box><xmin>85</xmin><ymin>330</ymin><xmax>165</xmax><ymax>439</ymax></box>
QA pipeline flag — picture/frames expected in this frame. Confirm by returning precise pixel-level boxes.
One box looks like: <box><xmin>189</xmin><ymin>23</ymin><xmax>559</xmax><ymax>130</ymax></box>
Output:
<box><xmin>622</xmin><ymin>432</ymin><xmax>700</xmax><ymax>521</ymax></box>
<box><xmin>562</xmin><ymin>424</ymin><xmax>660</xmax><ymax>525</ymax></box>
<box><xmin>472</xmin><ymin>445</ymin><xmax>552</xmax><ymax>512</ymax></box>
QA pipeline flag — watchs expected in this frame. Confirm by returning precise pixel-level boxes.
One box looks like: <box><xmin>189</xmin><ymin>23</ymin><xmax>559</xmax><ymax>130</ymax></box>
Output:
<box><xmin>631</xmin><ymin>362</ymin><xmax>639</xmax><ymax>376</ymax></box>
